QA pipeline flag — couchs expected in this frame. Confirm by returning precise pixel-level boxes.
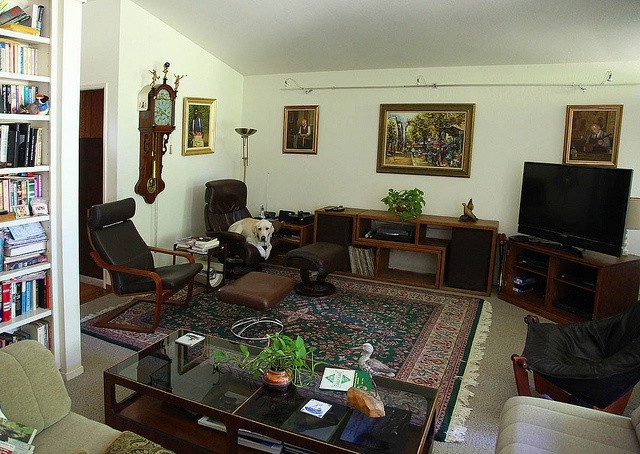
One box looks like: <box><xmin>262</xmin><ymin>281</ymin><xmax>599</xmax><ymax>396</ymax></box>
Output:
<box><xmin>495</xmin><ymin>395</ymin><xmax>640</xmax><ymax>454</ymax></box>
<box><xmin>0</xmin><ymin>339</ymin><xmax>174</xmax><ymax>453</ymax></box>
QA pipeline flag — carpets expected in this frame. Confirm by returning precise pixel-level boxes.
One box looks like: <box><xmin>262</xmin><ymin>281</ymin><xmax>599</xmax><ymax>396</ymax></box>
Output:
<box><xmin>80</xmin><ymin>261</ymin><xmax>492</xmax><ymax>442</ymax></box>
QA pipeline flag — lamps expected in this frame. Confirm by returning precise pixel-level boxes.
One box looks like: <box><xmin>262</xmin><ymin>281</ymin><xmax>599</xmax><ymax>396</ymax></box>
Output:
<box><xmin>601</xmin><ymin>69</ymin><xmax>615</xmax><ymax>85</ymax></box>
<box><xmin>622</xmin><ymin>197</ymin><xmax>640</xmax><ymax>257</ymax></box>
<box><xmin>415</xmin><ymin>74</ymin><xmax>427</xmax><ymax>87</ymax></box>
<box><xmin>283</xmin><ymin>77</ymin><xmax>303</xmax><ymax>90</ymax></box>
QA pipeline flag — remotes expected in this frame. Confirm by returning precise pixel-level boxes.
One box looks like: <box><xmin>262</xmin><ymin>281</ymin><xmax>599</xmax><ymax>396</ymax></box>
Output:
<box><xmin>324</xmin><ymin>206</ymin><xmax>335</xmax><ymax>211</ymax></box>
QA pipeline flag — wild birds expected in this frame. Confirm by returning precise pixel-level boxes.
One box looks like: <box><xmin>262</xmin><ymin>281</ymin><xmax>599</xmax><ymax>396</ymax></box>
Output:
<box><xmin>348</xmin><ymin>342</ymin><xmax>396</xmax><ymax>398</ymax></box>
<box><xmin>459</xmin><ymin>198</ymin><xmax>478</xmax><ymax>223</ymax></box>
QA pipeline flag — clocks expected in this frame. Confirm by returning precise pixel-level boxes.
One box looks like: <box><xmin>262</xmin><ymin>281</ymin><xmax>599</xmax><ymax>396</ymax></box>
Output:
<box><xmin>134</xmin><ymin>62</ymin><xmax>189</xmax><ymax>205</ymax></box>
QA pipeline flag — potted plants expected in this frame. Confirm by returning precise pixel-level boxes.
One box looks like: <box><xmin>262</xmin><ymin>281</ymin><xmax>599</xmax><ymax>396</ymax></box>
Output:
<box><xmin>380</xmin><ymin>188</ymin><xmax>425</xmax><ymax>221</ymax></box>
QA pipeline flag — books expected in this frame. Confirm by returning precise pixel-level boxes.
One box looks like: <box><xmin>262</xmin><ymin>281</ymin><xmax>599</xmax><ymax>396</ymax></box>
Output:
<box><xmin>0</xmin><ymin>84</ymin><xmax>38</xmax><ymax>114</ymax></box>
<box><xmin>348</xmin><ymin>246</ymin><xmax>374</xmax><ymax>276</ymax></box>
<box><xmin>0</xmin><ymin>276</ymin><xmax>50</xmax><ymax>323</ymax></box>
<box><xmin>191</xmin><ymin>237</ymin><xmax>220</xmax><ymax>252</ymax></box>
<box><xmin>0</xmin><ymin>38</ymin><xmax>38</xmax><ymax>75</ymax></box>
<box><xmin>1</xmin><ymin>320</ymin><xmax>50</xmax><ymax>350</ymax></box>
<box><xmin>0</xmin><ymin>0</ymin><xmax>44</xmax><ymax>36</ymax></box>
<box><xmin>319</xmin><ymin>368</ymin><xmax>372</xmax><ymax>392</ymax></box>
<box><xmin>0</xmin><ymin>123</ymin><xmax>49</xmax><ymax>167</ymax></box>
<box><xmin>0</xmin><ymin>173</ymin><xmax>42</xmax><ymax>213</ymax></box>
<box><xmin>4</xmin><ymin>221</ymin><xmax>49</xmax><ymax>271</ymax></box>
<box><xmin>0</xmin><ymin>417</ymin><xmax>37</xmax><ymax>454</ymax></box>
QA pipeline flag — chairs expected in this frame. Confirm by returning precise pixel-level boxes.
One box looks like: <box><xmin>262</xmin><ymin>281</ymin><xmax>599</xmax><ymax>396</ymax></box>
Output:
<box><xmin>86</xmin><ymin>198</ymin><xmax>203</xmax><ymax>334</ymax></box>
<box><xmin>204</xmin><ymin>179</ymin><xmax>282</xmax><ymax>278</ymax></box>
<box><xmin>510</xmin><ymin>301</ymin><xmax>638</xmax><ymax>417</ymax></box>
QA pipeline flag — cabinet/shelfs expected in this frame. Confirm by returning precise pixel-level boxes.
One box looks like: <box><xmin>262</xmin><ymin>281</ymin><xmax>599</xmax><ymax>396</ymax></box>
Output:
<box><xmin>497</xmin><ymin>237</ymin><xmax>640</xmax><ymax>326</ymax></box>
<box><xmin>313</xmin><ymin>205</ymin><xmax>499</xmax><ymax>298</ymax></box>
<box><xmin>0</xmin><ymin>0</ymin><xmax>59</xmax><ymax>369</ymax></box>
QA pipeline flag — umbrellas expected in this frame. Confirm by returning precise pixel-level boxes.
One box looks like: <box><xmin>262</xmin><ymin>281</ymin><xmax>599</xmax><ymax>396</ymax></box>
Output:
<box><xmin>0</xmin><ymin>233</ymin><xmax>4</xmax><ymax>272</ymax></box>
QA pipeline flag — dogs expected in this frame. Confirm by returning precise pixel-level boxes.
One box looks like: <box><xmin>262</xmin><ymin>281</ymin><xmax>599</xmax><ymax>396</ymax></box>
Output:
<box><xmin>228</xmin><ymin>217</ymin><xmax>275</xmax><ymax>260</ymax></box>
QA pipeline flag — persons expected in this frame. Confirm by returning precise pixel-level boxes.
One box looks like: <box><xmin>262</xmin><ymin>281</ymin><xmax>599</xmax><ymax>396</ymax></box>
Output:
<box><xmin>584</xmin><ymin>124</ymin><xmax>610</xmax><ymax>152</ymax></box>
<box><xmin>189</xmin><ymin>108</ymin><xmax>204</xmax><ymax>147</ymax></box>
<box><xmin>298</xmin><ymin>120</ymin><xmax>311</xmax><ymax>137</ymax></box>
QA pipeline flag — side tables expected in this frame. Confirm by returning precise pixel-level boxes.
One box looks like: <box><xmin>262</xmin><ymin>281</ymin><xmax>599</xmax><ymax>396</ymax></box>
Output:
<box><xmin>173</xmin><ymin>242</ymin><xmax>228</xmax><ymax>294</ymax></box>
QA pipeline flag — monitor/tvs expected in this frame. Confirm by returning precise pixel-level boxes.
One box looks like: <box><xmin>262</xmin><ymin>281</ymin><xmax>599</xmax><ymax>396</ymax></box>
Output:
<box><xmin>517</xmin><ymin>160</ymin><xmax>634</xmax><ymax>257</ymax></box>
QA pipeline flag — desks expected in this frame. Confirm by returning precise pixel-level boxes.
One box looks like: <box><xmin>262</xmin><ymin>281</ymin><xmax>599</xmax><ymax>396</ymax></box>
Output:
<box><xmin>272</xmin><ymin>220</ymin><xmax>314</xmax><ymax>248</ymax></box>
<box><xmin>103</xmin><ymin>328</ymin><xmax>440</xmax><ymax>454</ymax></box>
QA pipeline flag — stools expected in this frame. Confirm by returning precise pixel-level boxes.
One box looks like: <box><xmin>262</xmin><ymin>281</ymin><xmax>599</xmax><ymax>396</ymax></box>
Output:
<box><xmin>216</xmin><ymin>271</ymin><xmax>296</xmax><ymax>342</ymax></box>
<box><xmin>282</xmin><ymin>241</ymin><xmax>349</xmax><ymax>298</ymax></box>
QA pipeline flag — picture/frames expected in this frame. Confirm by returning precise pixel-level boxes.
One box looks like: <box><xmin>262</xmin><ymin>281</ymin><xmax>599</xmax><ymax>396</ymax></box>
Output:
<box><xmin>376</xmin><ymin>102</ymin><xmax>477</xmax><ymax>179</ymax></box>
<box><xmin>562</xmin><ymin>104</ymin><xmax>624</xmax><ymax>169</ymax></box>
<box><xmin>181</xmin><ymin>96</ymin><xmax>217</xmax><ymax>156</ymax></box>
<box><xmin>282</xmin><ymin>105</ymin><xmax>320</xmax><ymax>155</ymax></box>
